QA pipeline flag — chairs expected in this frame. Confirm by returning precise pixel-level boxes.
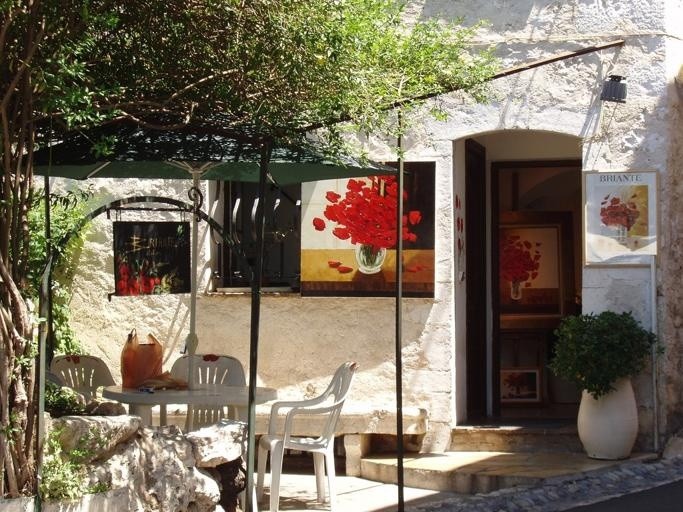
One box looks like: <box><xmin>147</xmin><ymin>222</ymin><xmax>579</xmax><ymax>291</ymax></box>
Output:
<box><xmin>257</xmin><ymin>362</ymin><xmax>360</xmax><ymax>512</ymax></box>
<box><xmin>160</xmin><ymin>354</ymin><xmax>250</xmax><ymax>435</ymax></box>
<box><xmin>45</xmin><ymin>355</ymin><xmax>128</xmax><ymax>417</ymax></box>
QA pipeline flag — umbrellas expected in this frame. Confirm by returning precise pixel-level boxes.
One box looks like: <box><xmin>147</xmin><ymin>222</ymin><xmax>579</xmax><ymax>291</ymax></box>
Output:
<box><xmin>0</xmin><ymin>110</ymin><xmax>411</xmax><ymax>512</ymax></box>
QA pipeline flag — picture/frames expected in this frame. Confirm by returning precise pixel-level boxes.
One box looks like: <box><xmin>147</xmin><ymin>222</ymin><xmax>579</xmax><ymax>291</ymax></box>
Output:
<box><xmin>583</xmin><ymin>169</ymin><xmax>661</xmax><ymax>268</ymax></box>
<box><xmin>500</xmin><ymin>369</ymin><xmax>541</xmax><ymax>403</ymax></box>
<box><xmin>499</xmin><ymin>223</ymin><xmax>565</xmax><ymax>319</ymax></box>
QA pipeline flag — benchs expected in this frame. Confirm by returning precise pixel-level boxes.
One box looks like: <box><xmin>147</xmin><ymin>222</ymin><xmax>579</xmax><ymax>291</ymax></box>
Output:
<box><xmin>152</xmin><ymin>399</ymin><xmax>433</xmax><ymax>475</ymax></box>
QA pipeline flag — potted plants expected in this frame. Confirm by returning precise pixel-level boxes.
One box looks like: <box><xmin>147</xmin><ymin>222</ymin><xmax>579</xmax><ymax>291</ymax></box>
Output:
<box><xmin>548</xmin><ymin>308</ymin><xmax>666</xmax><ymax>462</ymax></box>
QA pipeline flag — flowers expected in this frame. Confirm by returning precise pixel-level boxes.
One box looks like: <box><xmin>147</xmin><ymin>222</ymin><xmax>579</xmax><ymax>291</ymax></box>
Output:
<box><xmin>498</xmin><ymin>233</ymin><xmax>541</xmax><ymax>287</ymax></box>
<box><xmin>600</xmin><ymin>192</ymin><xmax>639</xmax><ymax>231</ymax></box>
<box><xmin>313</xmin><ymin>172</ymin><xmax>422</xmax><ymax>252</ymax></box>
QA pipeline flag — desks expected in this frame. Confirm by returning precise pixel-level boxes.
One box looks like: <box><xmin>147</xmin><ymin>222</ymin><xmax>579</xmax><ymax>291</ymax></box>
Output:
<box><xmin>103</xmin><ymin>384</ymin><xmax>278</xmax><ymax>434</ymax></box>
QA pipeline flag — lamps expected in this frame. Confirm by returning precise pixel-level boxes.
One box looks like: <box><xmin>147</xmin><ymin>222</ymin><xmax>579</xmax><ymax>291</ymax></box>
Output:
<box><xmin>599</xmin><ymin>73</ymin><xmax>628</xmax><ymax>103</ymax></box>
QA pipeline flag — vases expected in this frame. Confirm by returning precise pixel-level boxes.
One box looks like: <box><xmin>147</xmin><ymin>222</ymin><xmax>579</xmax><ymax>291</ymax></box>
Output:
<box><xmin>355</xmin><ymin>241</ymin><xmax>387</xmax><ymax>274</ymax></box>
<box><xmin>511</xmin><ymin>281</ymin><xmax>522</xmax><ymax>300</ymax></box>
<box><xmin>617</xmin><ymin>223</ymin><xmax>627</xmax><ymax>243</ymax></box>
